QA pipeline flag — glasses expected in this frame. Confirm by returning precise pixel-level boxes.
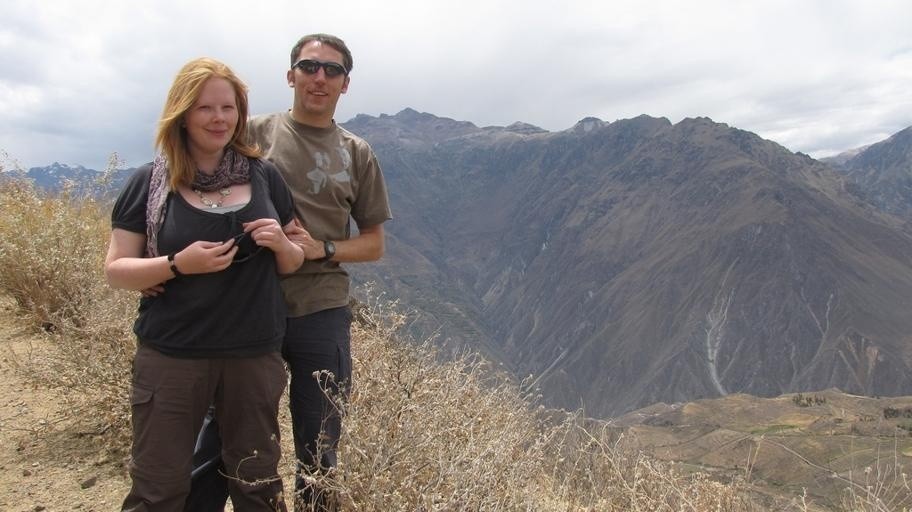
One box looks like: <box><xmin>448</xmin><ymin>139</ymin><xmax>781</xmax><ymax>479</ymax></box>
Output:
<box><xmin>291</xmin><ymin>59</ymin><xmax>347</xmax><ymax>78</ymax></box>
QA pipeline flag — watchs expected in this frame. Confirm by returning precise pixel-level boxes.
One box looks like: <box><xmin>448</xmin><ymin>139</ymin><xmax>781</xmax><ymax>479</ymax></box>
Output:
<box><xmin>322</xmin><ymin>237</ymin><xmax>336</xmax><ymax>263</ymax></box>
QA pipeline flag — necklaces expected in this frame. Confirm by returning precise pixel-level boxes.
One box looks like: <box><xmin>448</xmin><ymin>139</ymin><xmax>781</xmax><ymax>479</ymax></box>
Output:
<box><xmin>191</xmin><ymin>189</ymin><xmax>232</xmax><ymax>210</ymax></box>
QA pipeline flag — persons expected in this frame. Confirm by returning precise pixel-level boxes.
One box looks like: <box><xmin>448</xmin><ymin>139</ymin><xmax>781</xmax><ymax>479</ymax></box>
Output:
<box><xmin>134</xmin><ymin>33</ymin><xmax>396</xmax><ymax>510</ymax></box>
<box><xmin>101</xmin><ymin>57</ymin><xmax>306</xmax><ymax>512</ymax></box>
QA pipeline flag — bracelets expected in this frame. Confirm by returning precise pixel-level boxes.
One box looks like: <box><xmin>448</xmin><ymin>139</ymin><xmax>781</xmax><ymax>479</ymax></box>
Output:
<box><xmin>168</xmin><ymin>252</ymin><xmax>183</xmax><ymax>279</ymax></box>
